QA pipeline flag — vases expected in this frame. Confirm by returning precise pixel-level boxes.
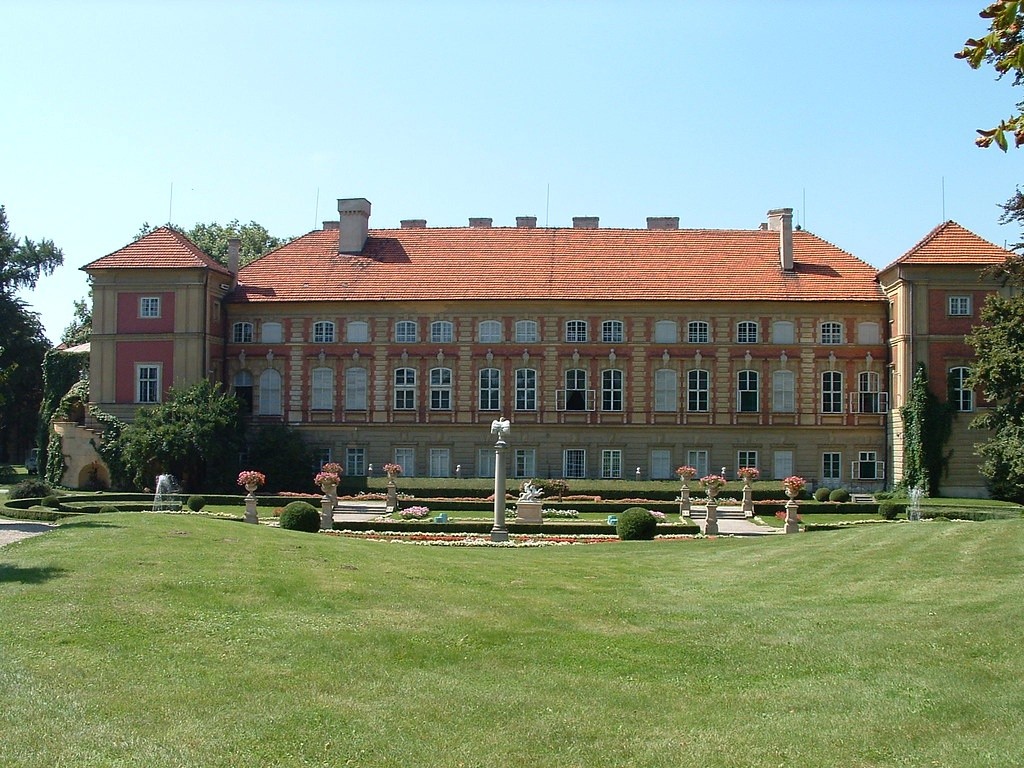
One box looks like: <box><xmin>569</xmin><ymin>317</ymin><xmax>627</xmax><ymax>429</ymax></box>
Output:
<box><xmin>388</xmin><ymin>473</ymin><xmax>398</xmax><ymax>483</ymax></box>
<box><xmin>785</xmin><ymin>488</ymin><xmax>799</xmax><ymax>503</ymax></box>
<box><xmin>321</xmin><ymin>484</ymin><xmax>336</xmax><ymax>498</ymax></box>
<box><xmin>705</xmin><ymin>487</ymin><xmax>719</xmax><ymax>502</ymax></box>
<box><xmin>245</xmin><ymin>484</ymin><xmax>257</xmax><ymax>496</ymax></box>
<box><xmin>680</xmin><ymin>474</ymin><xmax>691</xmax><ymax>487</ymax></box>
<box><xmin>742</xmin><ymin>478</ymin><xmax>754</xmax><ymax>487</ymax></box>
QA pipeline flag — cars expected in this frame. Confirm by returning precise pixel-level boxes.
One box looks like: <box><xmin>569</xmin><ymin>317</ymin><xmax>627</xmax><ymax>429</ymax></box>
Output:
<box><xmin>24</xmin><ymin>447</ymin><xmax>40</xmax><ymax>475</ymax></box>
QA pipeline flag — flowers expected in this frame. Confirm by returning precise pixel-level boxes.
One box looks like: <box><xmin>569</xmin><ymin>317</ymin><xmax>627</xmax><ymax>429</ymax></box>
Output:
<box><xmin>782</xmin><ymin>474</ymin><xmax>809</xmax><ymax>493</ymax></box>
<box><xmin>675</xmin><ymin>462</ymin><xmax>697</xmax><ymax>477</ymax></box>
<box><xmin>700</xmin><ymin>472</ymin><xmax>729</xmax><ymax>492</ymax></box>
<box><xmin>738</xmin><ymin>466</ymin><xmax>758</xmax><ymax>482</ymax></box>
<box><xmin>237</xmin><ymin>470</ymin><xmax>266</xmax><ymax>486</ymax></box>
<box><xmin>278</xmin><ymin>490</ymin><xmax>418</xmax><ymax>501</ymax></box>
<box><xmin>383</xmin><ymin>463</ymin><xmax>402</xmax><ymax>474</ymax></box>
<box><xmin>321</xmin><ymin>463</ymin><xmax>343</xmax><ymax>473</ymax></box>
<box><xmin>314</xmin><ymin>471</ymin><xmax>340</xmax><ymax>486</ymax></box>
<box><xmin>399</xmin><ymin>506</ymin><xmax>429</xmax><ymax>520</ymax></box>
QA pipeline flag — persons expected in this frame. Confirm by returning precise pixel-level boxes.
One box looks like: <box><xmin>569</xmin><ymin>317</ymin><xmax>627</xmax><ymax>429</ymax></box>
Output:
<box><xmin>519</xmin><ymin>479</ymin><xmax>544</xmax><ymax>500</ymax></box>
